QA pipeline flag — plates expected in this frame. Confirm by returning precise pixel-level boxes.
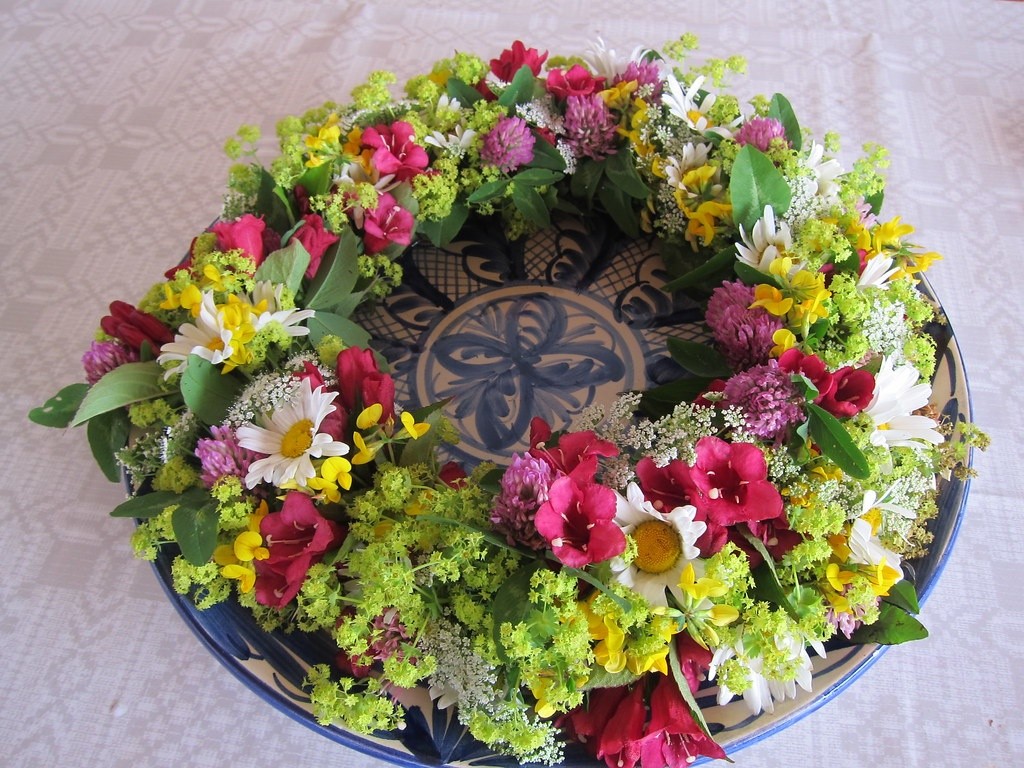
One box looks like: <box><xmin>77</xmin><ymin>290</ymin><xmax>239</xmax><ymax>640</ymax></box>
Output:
<box><xmin>122</xmin><ymin>215</ymin><xmax>974</xmax><ymax>768</ymax></box>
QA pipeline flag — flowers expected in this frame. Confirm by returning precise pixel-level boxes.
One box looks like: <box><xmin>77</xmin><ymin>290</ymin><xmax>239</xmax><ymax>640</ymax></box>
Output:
<box><xmin>26</xmin><ymin>27</ymin><xmax>990</xmax><ymax>768</ymax></box>
